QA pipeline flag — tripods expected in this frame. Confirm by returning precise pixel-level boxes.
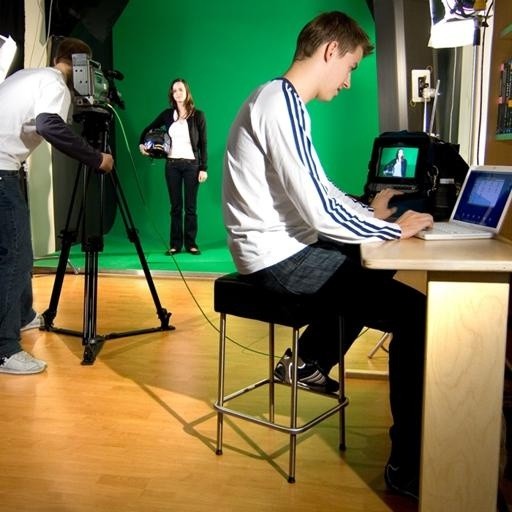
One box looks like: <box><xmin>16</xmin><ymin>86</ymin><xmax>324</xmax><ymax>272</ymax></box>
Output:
<box><xmin>39</xmin><ymin>107</ymin><xmax>175</xmax><ymax>366</ymax></box>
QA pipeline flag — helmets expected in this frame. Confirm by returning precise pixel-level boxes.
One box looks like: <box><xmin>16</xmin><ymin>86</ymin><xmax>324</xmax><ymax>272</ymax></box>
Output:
<box><xmin>144</xmin><ymin>129</ymin><xmax>171</xmax><ymax>159</ymax></box>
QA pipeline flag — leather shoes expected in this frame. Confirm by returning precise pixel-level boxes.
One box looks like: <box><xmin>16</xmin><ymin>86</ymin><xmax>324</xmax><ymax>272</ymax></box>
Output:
<box><xmin>166</xmin><ymin>248</ymin><xmax>181</xmax><ymax>255</ymax></box>
<box><xmin>187</xmin><ymin>247</ymin><xmax>200</xmax><ymax>255</ymax></box>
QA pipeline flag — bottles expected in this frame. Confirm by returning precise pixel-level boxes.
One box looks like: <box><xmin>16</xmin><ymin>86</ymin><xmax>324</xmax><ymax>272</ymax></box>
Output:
<box><xmin>438</xmin><ymin>178</ymin><xmax>456</xmax><ymax>222</ymax></box>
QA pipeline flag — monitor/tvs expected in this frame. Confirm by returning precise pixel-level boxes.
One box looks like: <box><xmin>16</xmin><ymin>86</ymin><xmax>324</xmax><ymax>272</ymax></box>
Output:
<box><xmin>359</xmin><ymin>131</ymin><xmax>469</xmax><ymax>221</ymax></box>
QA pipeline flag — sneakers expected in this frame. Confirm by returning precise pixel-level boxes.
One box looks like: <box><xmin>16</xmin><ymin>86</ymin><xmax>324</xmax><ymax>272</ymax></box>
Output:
<box><xmin>19</xmin><ymin>312</ymin><xmax>55</xmax><ymax>331</ymax></box>
<box><xmin>0</xmin><ymin>349</ymin><xmax>46</xmax><ymax>375</ymax></box>
<box><xmin>383</xmin><ymin>455</ymin><xmax>420</xmax><ymax>501</ymax></box>
<box><xmin>275</xmin><ymin>347</ymin><xmax>340</xmax><ymax>392</ymax></box>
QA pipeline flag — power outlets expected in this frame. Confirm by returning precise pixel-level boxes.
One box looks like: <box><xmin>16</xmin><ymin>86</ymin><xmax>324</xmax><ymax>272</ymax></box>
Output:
<box><xmin>411</xmin><ymin>68</ymin><xmax>432</xmax><ymax>103</ymax></box>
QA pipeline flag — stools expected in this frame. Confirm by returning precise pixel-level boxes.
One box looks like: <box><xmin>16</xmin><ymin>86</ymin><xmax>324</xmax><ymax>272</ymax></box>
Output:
<box><xmin>215</xmin><ymin>273</ymin><xmax>348</xmax><ymax>484</ymax></box>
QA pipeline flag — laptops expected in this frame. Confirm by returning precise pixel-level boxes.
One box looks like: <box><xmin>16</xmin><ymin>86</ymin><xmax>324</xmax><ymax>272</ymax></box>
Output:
<box><xmin>413</xmin><ymin>165</ymin><xmax>512</xmax><ymax>240</ymax></box>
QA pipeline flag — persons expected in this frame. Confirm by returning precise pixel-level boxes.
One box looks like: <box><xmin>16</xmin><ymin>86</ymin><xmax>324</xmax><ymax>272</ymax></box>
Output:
<box><xmin>220</xmin><ymin>11</ymin><xmax>434</xmax><ymax>505</ymax></box>
<box><xmin>383</xmin><ymin>149</ymin><xmax>407</xmax><ymax>178</ymax></box>
<box><xmin>138</xmin><ymin>79</ymin><xmax>208</xmax><ymax>256</ymax></box>
<box><xmin>0</xmin><ymin>38</ymin><xmax>114</xmax><ymax>375</ymax></box>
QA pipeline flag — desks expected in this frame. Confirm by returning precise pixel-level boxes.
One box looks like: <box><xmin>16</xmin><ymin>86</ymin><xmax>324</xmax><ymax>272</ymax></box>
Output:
<box><xmin>361</xmin><ymin>238</ymin><xmax>512</xmax><ymax>512</ymax></box>
